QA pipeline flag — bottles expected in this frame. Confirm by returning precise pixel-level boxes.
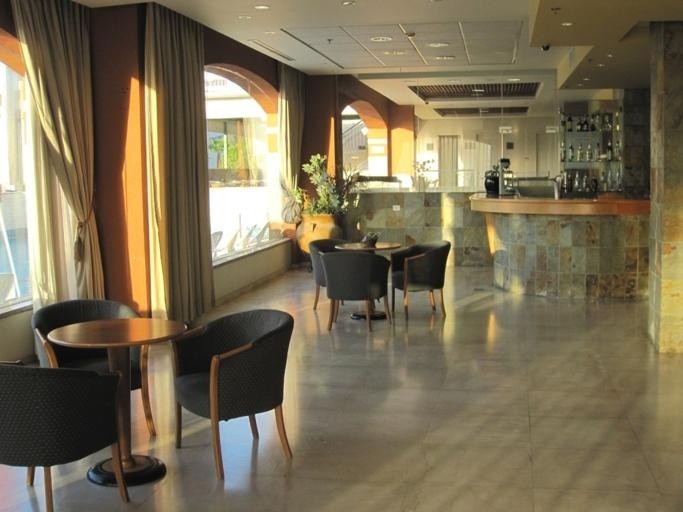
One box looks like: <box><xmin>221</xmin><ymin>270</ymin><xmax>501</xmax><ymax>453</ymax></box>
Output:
<box><xmin>599</xmin><ymin>171</ymin><xmax>607</xmax><ymax>192</ymax></box>
<box><xmin>577</xmin><ymin>143</ymin><xmax>584</xmax><ymax>162</ymax></box>
<box><xmin>614</xmin><ymin>169</ymin><xmax>623</xmax><ymax>192</ymax></box>
<box><xmin>606</xmin><ymin>139</ymin><xmax>613</xmax><ymax>161</ymax></box>
<box><xmin>576</xmin><ymin>118</ymin><xmax>582</xmax><ymax>132</ymax></box>
<box><xmin>562</xmin><ymin>171</ymin><xmax>569</xmax><ymax>194</ymax></box>
<box><xmin>594</xmin><ymin>142</ymin><xmax>601</xmax><ymax>161</ymax></box>
<box><xmin>586</xmin><ymin>143</ymin><xmax>593</xmax><ymax>161</ymax></box>
<box><xmin>590</xmin><ymin>175</ymin><xmax>598</xmax><ymax>192</ymax></box>
<box><xmin>568</xmin><ymin>143</ymin><xmax>574</xmax><ymax>161</ymax></box>
<box><xmin>583</xmin><ymin>116</ymin><xmax>589</xmax><ymax>131</ymax></box>
<box><xmin>560</xmin><ymin>141</ymin><xmax>566</xmax><ymax>161</ymax></box>
<box><xmin>574</xmin><ymin>170</ymin><xmax>580</xmax><ymax>191</ymax></box>
<box><xmin>607</xmin><ymin>169</ymin><xmax>613</xmax><ymax>191</ymax></box>
<box><xmin>567</xmin><ymin>113</ymin><xmax>573</xmax><ymax>132</ymax></box>
<box><xmin>560</xmin><ymin>111</ymin><xmax>566</xmax><ymax>131</ymax></box>
<box><xmin>582</xmin><ymin>175</ymin><xmax>590</xmax><ymax>193</ymax></box>
<box><xmin>614</xmin><ymin>140</ymin><xmax>620</xmax><ymax>161</ymax></box>
<box><xmin>590</xmin><ymin>116</ymin><xmax>595</xmax><ymax>130</ymax></box>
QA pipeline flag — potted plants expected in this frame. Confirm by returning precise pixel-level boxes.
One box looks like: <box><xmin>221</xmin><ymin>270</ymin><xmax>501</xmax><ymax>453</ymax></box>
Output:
<box><xmin>285</xmin><ymin>153</ymin><xmax>362</xmax><ymax>254</ymax></box>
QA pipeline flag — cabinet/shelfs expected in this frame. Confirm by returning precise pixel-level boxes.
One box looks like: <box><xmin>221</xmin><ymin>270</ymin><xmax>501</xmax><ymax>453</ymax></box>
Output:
<box><xmin>556</xmin><ymin>100</ymin><xmax>624</xmax><ymax>193</ymax></box>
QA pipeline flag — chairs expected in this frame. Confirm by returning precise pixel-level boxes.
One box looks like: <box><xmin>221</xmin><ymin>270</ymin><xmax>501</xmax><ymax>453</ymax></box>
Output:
<box><xmin>0</xmin><ymin>360</ymin><xmax>131</xmax><ymax>512</ymax></box>
<box><xmin>318</xmin><ymin>251</ymin><xmax>392</xmax><ymax>332</ymax></box>
<box><xmin>308</xmin><ymin>239</ymin><xmax>346</xmax><ymax>310</ymax></box>
<box><xmin>172</xmin><ymin>309</ymin><xmax>294</xmax><ymax>480</ymax></box>
<box><xmin>392</xmin><ymin>241</ymin><xmax>451</xmax><ymax>321</ymax></box>
<box><xmin>32</xmin><ymin>300</ymin><xmax>158</xmax><ymax>437</ymax></box>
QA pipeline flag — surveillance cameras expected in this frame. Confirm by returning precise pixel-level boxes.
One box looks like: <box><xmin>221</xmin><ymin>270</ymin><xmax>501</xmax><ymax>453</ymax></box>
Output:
<box><xmin>540</xmin><ymin>46</ymin><xmax>550</xmax><ymax>51</ymax></box>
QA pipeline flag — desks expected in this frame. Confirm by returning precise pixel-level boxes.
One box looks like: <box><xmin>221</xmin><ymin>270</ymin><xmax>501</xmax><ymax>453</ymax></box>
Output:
<box><xmin>47</xmin><ymin>319</ymin><xmax>187</xmax><ymax>488</ymax></box>
<box><xmin>334</xmin><ymin>242</ymin><xmax>401</xmax><ymax>321</ymax></box>
<box><xmin>469</xmin><ymin>192</ymin><xmax>650</xmax><ymax>303</ymax></box>
